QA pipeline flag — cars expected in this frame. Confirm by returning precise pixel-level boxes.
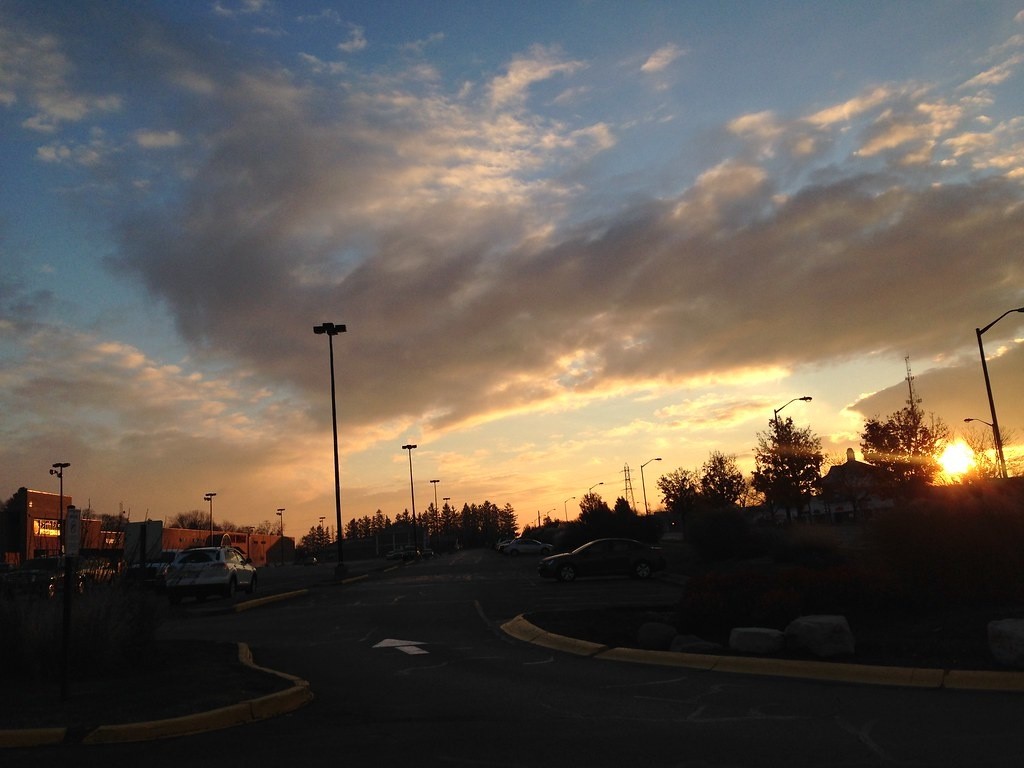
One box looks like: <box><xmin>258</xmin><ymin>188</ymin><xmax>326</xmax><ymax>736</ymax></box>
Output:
<box><xmin>126</xmin><ymin>549</ymin><xmax>185</xmax><ymax>590</ymax></box>
<box><xmin>304</xmin><ymin>556</ymin><xmax>318</xmax><ymax>566</ymax></box>
<box><xmin>495</xmin><ymin>538</ymin><xmax>554</xmax><ymax>557</ymax></box>
<box><xmin>0</xmin><ymin>557</ymin><xmax>85</xmax><ymax>595</ymax></box>
<box><xmin>164</xmin><ymin>546</ymin><xmax>257</xmax><ymax>603</ymax></box>
<box><xmin>537</xmin><ymin>539</ymin><xmax>658</xmax><ymax>579</ymax></box>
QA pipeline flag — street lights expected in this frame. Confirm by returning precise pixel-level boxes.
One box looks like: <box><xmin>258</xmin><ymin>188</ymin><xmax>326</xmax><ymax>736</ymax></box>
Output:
<box><xmin>443</xmin><ymin>497</ymin><xmax>449</xmax><ymax>506</ymax></box>
<box><xmin>313</xmin><ymin>321</ymin><xmax>350</xmax><ymax>583</ymax></box>
<box><xmin>589</xmin><ymin>482</ymin><xmax>604</xmax><ymax>495</ymax></box>
<box><xmin>429</xmin><ymin>479</ymin><xmax>441</xmax><ymax>554</ymax></box>
<box><xmin>965</xmin><ymin>418</ymin><xmax>1002</xmax><ymax>479</ymax></box>
<box><xmin>277</xmin><ymin>508</ymin><xmax>286</xmax><ymax>565</ymax></box>
<box><xmin>641</xmin><ymin>458</ymin><xmax>661</xmax><ymax>515</ymax></box>
<box><xmin>975</xmin><ymin>306</ymin><xmax>1024</xmax><ymax>477</ymax></box>
<box><xmin>204</xmin><ymin>494</ymin><xmax>217</xmax><ymax>546</ymax></box>
<box><xmin>773</xmin><ymin>396</ymin><xmax>812</xmax><ymax>524</ymax></box>
<box><xmin>402</xmin><ymin>445</ymin><xmax>418</xmax><ymax>560</ymax></box>
<box><xmin>50</xmin><ymin>464</ymin><xmax>70</xmax><ymax>567</ymax></box>
<box><xmin>564</xmin><ymin>497</ymin><xmax>574</xmax><ymax>522</ymax></box>
<box><xmin>319</xmin><ymin>517</ymin><xmax>324</xmax><ymax>536</ymax></box>
